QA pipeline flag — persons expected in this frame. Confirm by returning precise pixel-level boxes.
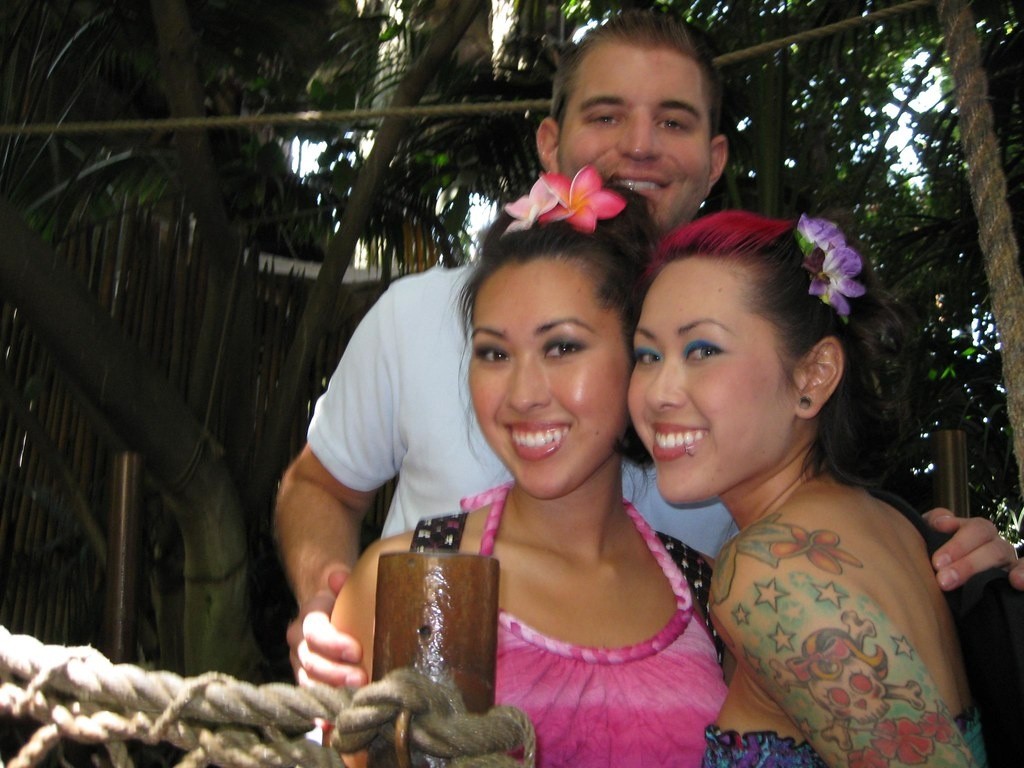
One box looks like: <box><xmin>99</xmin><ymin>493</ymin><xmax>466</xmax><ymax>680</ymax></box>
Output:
<box><xmin>274</xmin><ymin>10</ymin><xmax>1024</xmax><ymax>768</ymax></box>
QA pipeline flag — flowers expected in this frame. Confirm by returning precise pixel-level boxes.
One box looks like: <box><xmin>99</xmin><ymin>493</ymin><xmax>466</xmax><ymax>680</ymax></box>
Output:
<box><xmin>794</xmin><ymin>214</ymin><xmax>869</xmax><ymax>325</ymax></box>
<box><xmin>504</xmin><ymin>164</ymin><xmax>627</xmax><ymax>235</ymax></box>
